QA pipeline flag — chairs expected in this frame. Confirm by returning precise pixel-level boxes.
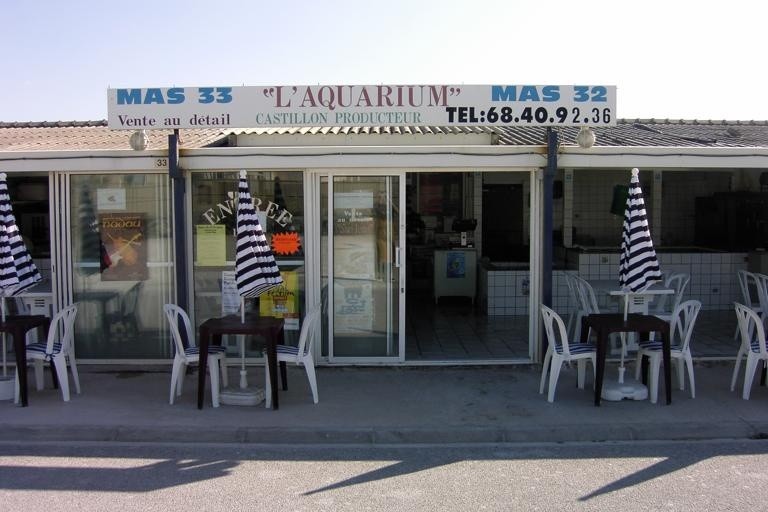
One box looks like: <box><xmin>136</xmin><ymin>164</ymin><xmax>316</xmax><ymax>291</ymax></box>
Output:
<box><xmin>13</xmin><ymin>301</ymin><xmax>80</xmax><ymax>407</ymax></box>
<box><xmin>108</xmin><ymin>281</ymin><xmax>143</xmax><ymax>347</ymax></box>
<box><xmin>539</xmin><ymin>270</ymin><xmax>768</xmax><ymax>407</ymax></box>
<box><xmin>262</xmin><ymin>301</ymin><xmax>320</xmax><ymax>411</ymax></box>
<box><xmin>163</xmin><ymin>301</ymin><xmax>231</xmax><ymax>409</ymax></box>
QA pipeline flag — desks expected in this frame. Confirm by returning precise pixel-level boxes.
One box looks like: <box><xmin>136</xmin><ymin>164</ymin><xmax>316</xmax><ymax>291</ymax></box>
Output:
<box><xmin>72</xmin><ymin>291</ymin><xmax>121</xmax><ymax>353</ymax></box>
<box><xmin>1</xmin><ymin>312</ymin><xmax>60</xmax><ymax>407</ymax></box>
<box><xmin>193</xmin><ymin>310</ymin><xmax>287</xmax><ymax>411</ymax></box>
<box><xmin>13</xmin><ymin>279</ymin><xmax>54</xmax><ymax>315</ymax></box>
<box><xmin>433</xmin><ymin>248</ymin><xmax>478</xmax><ymax>307</ymax></box>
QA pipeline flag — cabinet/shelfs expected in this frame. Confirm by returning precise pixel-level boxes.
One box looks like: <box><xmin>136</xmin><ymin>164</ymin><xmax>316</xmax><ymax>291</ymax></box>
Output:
<box><xmin>411</xmin><ymin>173</ymin><xmax>463</xmax><ymax>215</ymax></box>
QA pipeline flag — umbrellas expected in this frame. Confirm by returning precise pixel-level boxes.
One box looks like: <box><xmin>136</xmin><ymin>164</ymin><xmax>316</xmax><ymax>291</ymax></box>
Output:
<box><xmin>618</xmin><ymin>167</ymin><xmax>664</xmax><ymax>368</ymax></box>
<box><xmin>234</xmin><ymin>170</ymin><xmax>284</xmax><ymax>369</ymax></box>
<box><xmin>0</xmin><ymin>171</ymin><xmax>43</xmax><ymax>376</ymax></box>
